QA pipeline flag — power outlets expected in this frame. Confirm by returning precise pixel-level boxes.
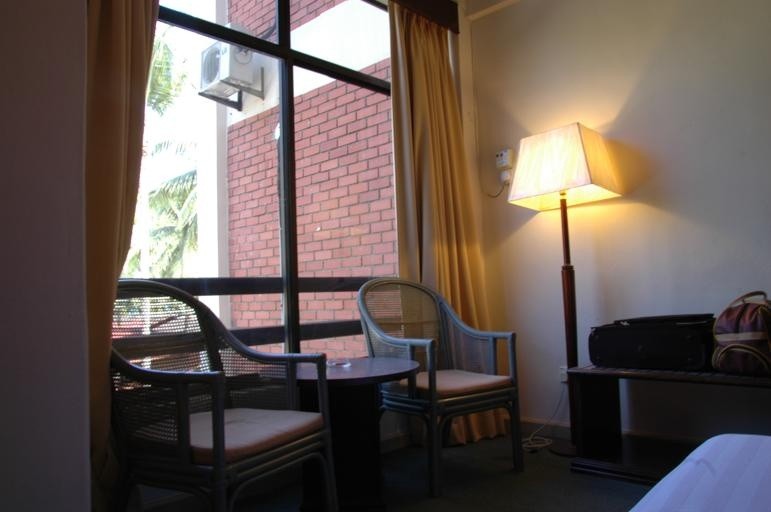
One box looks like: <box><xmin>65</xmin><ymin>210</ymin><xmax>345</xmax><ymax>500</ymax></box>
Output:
<box><xmin>560</xmin><ymin>367</ymin><xmax>568</xmax><ymax>383</ymax></box>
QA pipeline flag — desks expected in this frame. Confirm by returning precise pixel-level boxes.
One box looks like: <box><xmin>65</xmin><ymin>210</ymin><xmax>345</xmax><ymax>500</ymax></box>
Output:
<box><xmin>566</xmin><ymin>364</ymin><xmax>771</xmax><ymax>488</ymax></box>
<box><xmin>258</xmin><ymin>356</ymin><xmax>419</xmax><ymax>510</ymax></box>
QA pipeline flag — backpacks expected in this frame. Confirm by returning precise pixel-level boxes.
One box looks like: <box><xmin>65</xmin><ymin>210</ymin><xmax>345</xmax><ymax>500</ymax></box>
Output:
<box><xmin>711</xmin><ymin>291</ymin><xmax>771</xmax><ymax>377</ymax></box>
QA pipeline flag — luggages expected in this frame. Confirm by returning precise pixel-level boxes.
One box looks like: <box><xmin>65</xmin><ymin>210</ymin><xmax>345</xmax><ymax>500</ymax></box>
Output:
<box><xmin>588</xmin><ymin>312</ymin><xmax>715</xmax><ymax>372</ymax></box>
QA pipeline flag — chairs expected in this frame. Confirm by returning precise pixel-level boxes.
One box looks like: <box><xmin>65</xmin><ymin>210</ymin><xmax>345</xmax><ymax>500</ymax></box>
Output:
<box><xmin>114</xmin><ymin>280</ymin><xmax>339</xmax><ymax>510</ymax></box>
<box><xmin>358</xmin><ymin>277</ymin><xmax>520</xmax><ymax>500</ymax></box>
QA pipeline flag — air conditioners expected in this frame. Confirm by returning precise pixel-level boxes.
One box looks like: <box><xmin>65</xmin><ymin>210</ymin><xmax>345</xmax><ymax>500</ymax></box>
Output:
<box><xmin>198</xmin><ymin>23</ymin><xmax>263</xmax><ymax>100</ymax></box>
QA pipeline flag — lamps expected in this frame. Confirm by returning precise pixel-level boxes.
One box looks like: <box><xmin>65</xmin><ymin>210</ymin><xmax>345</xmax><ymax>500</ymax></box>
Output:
<box><xmin>506</xmin><ymin>121</ymin><xmax>623</xmax><ymax>448</ymax></box>
